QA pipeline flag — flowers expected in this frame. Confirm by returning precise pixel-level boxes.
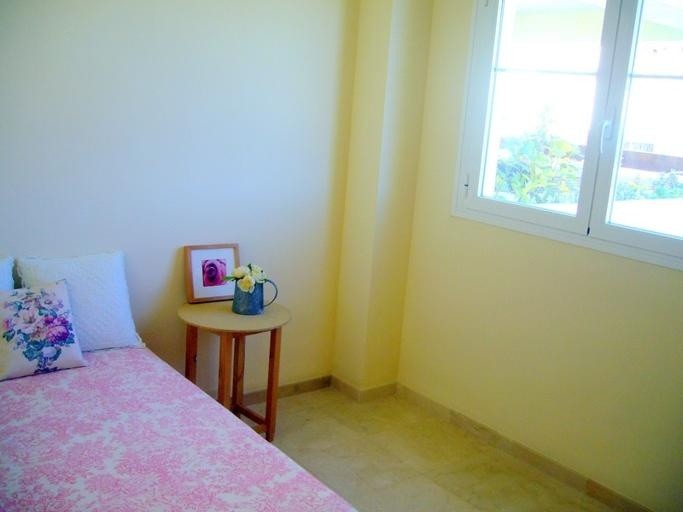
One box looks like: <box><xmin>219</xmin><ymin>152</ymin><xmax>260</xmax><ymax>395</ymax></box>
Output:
<box><xmin>222</xmin><ymin>263</ymin><xmax>268</xmax><ymax>295</ymax></box>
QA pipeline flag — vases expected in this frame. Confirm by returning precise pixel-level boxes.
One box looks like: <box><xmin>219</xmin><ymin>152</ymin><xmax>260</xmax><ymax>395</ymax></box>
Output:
<box><xmin>230</xmin><ymin>279</ymin><xmax>278</xmax><ymax>315</ymax></box>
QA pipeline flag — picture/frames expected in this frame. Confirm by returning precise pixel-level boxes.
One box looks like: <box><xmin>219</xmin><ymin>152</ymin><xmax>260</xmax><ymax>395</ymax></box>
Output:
<box><xmin>183</xmin><ymin>243</ymin><xmax>241</xmax><ymax>305</ymax></box>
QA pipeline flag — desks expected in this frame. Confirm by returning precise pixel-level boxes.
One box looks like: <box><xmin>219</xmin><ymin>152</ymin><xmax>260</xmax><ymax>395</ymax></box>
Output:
<box><xmin>180</xmin><ymin>302</ymin><xmax>291</xmax><ymax>446</ymax></box>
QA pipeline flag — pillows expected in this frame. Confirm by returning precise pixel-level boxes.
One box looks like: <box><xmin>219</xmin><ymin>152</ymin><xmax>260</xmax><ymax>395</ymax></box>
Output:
<box><xmin>0</xmin><ymin>247</ymin><xmax>145</xmax><ymax>382</ymax></box>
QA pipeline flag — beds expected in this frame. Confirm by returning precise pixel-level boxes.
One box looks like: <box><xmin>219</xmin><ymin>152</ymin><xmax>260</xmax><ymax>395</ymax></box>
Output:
<box><xmin>0</xmin><ymin>347</ymin><xmax>361</xmax><ymax>510</ymax></box>
